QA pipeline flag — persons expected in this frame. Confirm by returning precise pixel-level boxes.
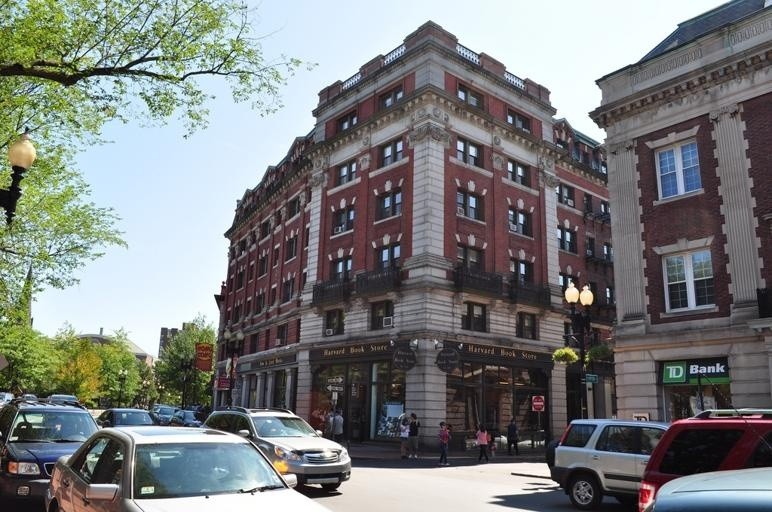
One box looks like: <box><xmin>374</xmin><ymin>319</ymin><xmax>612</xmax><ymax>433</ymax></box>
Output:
<box><xmin>507</xmin><ymin>419</ymin><xmax>522</xmax><ymax>455</ymax></box>
<box><xmin>476</xmin><ymin>425</ymin><xmax>490</xmax><ymax>464</ymax></box>
<box><xmin>320</xmin><ymin>408</ymin><xmax>422</xmax><ymax>460</ymax></box>
<box><xmin>187</xmin><ymin>402</ymin><xmax>211</xmax><ymax>421</ymax></box>
<box><xmin>436</xmin><ymin>422</ymin><xmax>452</xmax><ymax>467</ymax></box>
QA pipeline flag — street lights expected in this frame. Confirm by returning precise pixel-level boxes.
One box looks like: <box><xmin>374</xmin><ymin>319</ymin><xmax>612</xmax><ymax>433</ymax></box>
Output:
<box><xmin>142</xmin><ymin>378</ymin><xmax>150</xmax><ymax>411</ymax></box>
<box><xmin>223</xmin><ymin>329</ymin><xmax>244</xmax><ymax>406</ymax></box>
<box><xmin>117</xmin><ymin>369</ymin><xmax>128</xmax><ymax>408</ymax></box>
<box><xmin>180</xmin><ymin>353</ymin><xmax>193</xmax><ymax>411</ymax></box>
<box><xmin>0</xmin><ymin>132</ymin><xmax>37</xmax><ymax>223</ymax></box>
<box><xmin>156</xmin><ymin>377</ymin><xmax>164</xmax><ymax>405</ymax></box>
<box><xmin>565</xmin><ymin>282</ymin><xmax>595</xmax><ymax>421</ymax></box>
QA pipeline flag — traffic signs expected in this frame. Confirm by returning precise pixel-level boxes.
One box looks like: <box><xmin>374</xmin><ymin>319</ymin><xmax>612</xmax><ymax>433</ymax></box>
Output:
<box><xmin>326</xmin><ymin>384</ymin><xmax>345</xmax><ymax>393</ymax></box>
<box><xmin>327</xmin><ymin>376</ymin><xmax>344</xmax><ymax>384</ymax></box>
<box><xmin>585</xmin><ymin>374</ymin><xmax>599</xmax><ymax>385</ymax></box>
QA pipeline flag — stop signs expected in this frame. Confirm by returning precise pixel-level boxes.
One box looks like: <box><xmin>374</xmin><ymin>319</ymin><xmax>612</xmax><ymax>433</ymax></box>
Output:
<box><xmin>532</xmin><ymin>396</ymin><xmax>544</xmax><ymax>412</ymax></box>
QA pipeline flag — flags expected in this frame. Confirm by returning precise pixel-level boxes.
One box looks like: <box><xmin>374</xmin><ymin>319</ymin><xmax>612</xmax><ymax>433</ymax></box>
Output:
<box><xmin>196</xmin><ymin>342</ymin><xmax>213</xmax><ymax>372</ymax></box>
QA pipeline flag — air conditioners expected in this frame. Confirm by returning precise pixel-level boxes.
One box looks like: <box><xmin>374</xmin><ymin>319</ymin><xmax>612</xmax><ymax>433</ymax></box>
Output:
<box><xmin>312</xmin><ymin>153</ymin><xmax>329</xmax><ymax>173</ymax></box>
<box><xmin>587</xmin><ymin>249</ymin><xmax>593</xmax><ymax>255</ymax></box>
<box><xmin>567</xmin><ymin>198</ymin><xmax>575</xmax><ymax>207</ymax></box>
<box><xmin>457</xmin><ymin>206</ymin><xmax>465</xmax><ymax>216</ymax></box>
<box><xmin>334</xmin><ymin>226</ymin><xmax>341</xmax><ymax>234</ymax></box>
<box><xmin>382</xmin><ymin>316</ymin><xmax>393</xmax><ymax>328</ymax></box>
<box><xmin>357</xmin><ymin>135</ymin><xmax>370</xmax><ymax>148</ymax></box>
<box><xmin>509</xmin><ymin>224</ymin><xmax>517</xmax><ymax>232</ymax></box>
<box><xmin>409</xmin><ymin>105</ymin><xmax>451</xmax><ymax>124</ymax></box>
<box><xmin>494</xmin><ymin>135</ymin><xmax>506</xmax><ymax>151</ymax></box>
<box><xmin>540</xmin><ymin>155</ymin><xmax>555</xmax><ymax>173</ymax></box>
<box><xmin>325</xmin><ymin>329</ymin><xmax>334</xmax><ymax>336</ymax></box>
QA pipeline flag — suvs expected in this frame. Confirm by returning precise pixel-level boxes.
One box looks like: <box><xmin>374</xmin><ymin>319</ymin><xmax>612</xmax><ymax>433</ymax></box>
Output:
<box><xmin>633</xmin><ymin>408</ymin><xmax>772</xmax><ymax>512</ymax></box>
<box><xmin>545</xmin><ymin>418</ymin><xmax>671</xmax><ymax>510</ymax></box>
<box><xmin>203</xmin><ymin>407</ymin><xmax>352</xmax><ymax>492</ymax></box>
<box><xmin>0</xmin><ymin>400</ymin><xmax>99</xmax><ymax>512</ymax></box>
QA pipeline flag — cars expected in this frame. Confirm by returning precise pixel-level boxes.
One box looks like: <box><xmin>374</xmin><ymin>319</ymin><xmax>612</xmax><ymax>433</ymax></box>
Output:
<box><xmin>0</xmin><ymin>387</ymin><xmax>209</xmax><ymax>427</ymax></box>
<box><xmin>652</xmin><ymin>466</ymin><xmax>771</xmax><ymax>512</ymax></box>
<box><xmin>44</xmin><ymin>425</ymin><xmax>333</xmax><ymax>512</ymax></box>
